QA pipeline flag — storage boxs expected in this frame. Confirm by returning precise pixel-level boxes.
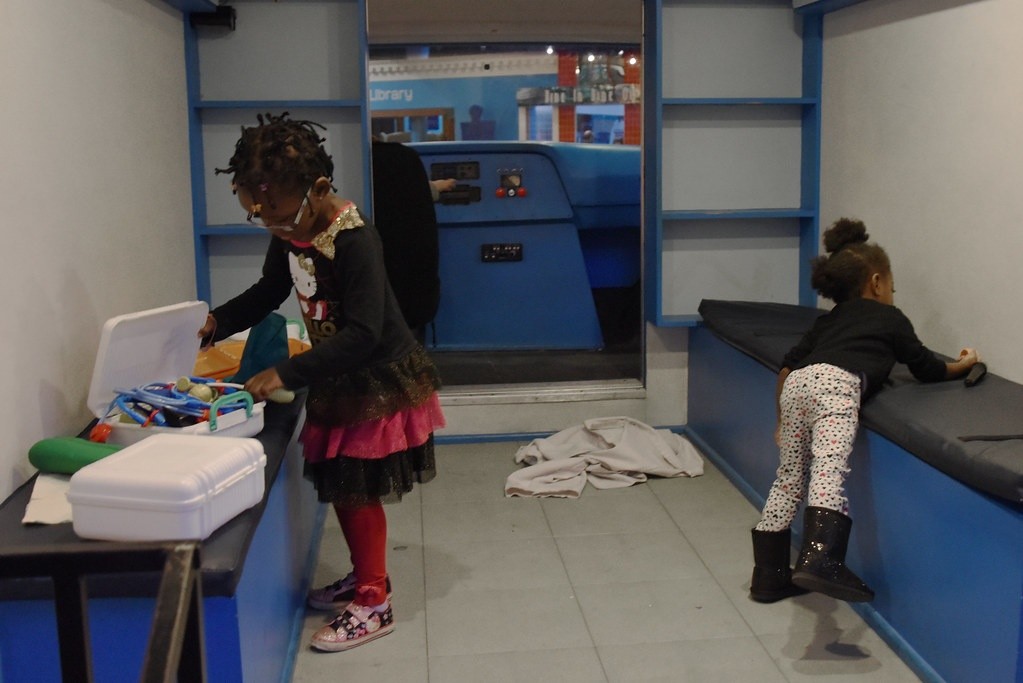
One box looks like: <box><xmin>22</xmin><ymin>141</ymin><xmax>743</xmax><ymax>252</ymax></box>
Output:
<box><xmin>84</xmin><ymin>300</ymin><xmax>266</xmax><ymax>451</ymax></box>
<box><xmin>67</xmin><ymin>433</ymin><xmax>267</xmax><ymax>541</ymax></box>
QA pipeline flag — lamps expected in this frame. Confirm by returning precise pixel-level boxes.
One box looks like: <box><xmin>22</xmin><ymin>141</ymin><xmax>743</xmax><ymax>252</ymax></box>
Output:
<box><xmin>545</xmin><ymin>46</ymin><xmax>554</xmax><ymax>54</ymax></box>
<box><xmin>587</xmin><ymin>52</ymin><xmax>595</xmax><ymax>61</ymax></box>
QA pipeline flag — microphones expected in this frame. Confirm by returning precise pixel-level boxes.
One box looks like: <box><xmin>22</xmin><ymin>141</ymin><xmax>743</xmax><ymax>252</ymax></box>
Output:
<box><xmin>963</xmin><ymin>362</ymin><xmax>986</xmax><ymax>387</ymax></box>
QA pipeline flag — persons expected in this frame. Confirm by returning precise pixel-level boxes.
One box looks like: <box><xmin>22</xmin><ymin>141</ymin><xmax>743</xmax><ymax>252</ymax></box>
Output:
<box><xmin>750</xmin><ymin>218</ymin><xmax>978</xmax><ymax>604</ymax></box>
<box><xmin>163</xmin><ymin>111</ymin><xmax>446</xmax><ymax>652</ymax></box>
<box><xmin>371</xmin><ymin>133</ymin><xmax>456</xmax><ymax>203</ymax></box>
<box><xmin>369</xmin><ymin>119</ymin><xmax>441</xmax><ymax>349</ymax></box>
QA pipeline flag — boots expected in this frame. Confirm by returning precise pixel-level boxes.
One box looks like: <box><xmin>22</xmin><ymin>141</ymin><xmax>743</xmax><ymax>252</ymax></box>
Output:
<box><xmin>749</xmin><ymin>526</ymin><xmax>811</xmax><ymax>603</ymax></box>
<box><xmin>791</xmin><ymin>505</ymin><xmax>875</xmax><ymax>602</ymax></box>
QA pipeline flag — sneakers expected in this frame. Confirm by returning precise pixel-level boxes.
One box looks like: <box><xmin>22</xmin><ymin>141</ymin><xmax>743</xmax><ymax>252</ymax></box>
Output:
<box><xmin>309</xmin><ymin>572</ymin><xmax>392</xmax><ymax>610</ymax></box>
<box><xmin>310</xmin><ymin>600</ymin><xmax>394</xmax><ymax>651</ymax></box>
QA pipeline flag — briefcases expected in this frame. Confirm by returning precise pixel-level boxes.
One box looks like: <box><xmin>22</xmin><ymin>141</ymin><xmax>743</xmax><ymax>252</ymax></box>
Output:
<box><xmin>64</xmin><ymin>432</ymin><xmax>268</xmax><ymax>541</ymax></box>
<box><xmin>83</xmin><ymin>298</ymin><xmax>268</xmax><ymax>455</ymax></box>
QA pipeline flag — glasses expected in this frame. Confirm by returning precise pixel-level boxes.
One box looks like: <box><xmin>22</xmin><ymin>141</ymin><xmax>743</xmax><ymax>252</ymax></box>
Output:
<box><xmin>245</xmin><ymin>185</ymin><xmax>313</xmax><ymax>231</ymax></box>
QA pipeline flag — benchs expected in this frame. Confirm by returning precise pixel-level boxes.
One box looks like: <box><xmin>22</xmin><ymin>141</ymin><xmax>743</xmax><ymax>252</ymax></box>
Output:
<box><xmin>685</xmin><ymin>297</ymin><xmax>1023</xmax><ymax>683</ymax></box>
<box><xmin>0</xmin><ymin>383</ymin><xmax>327</xmax><ymax>683</ymax></box>
